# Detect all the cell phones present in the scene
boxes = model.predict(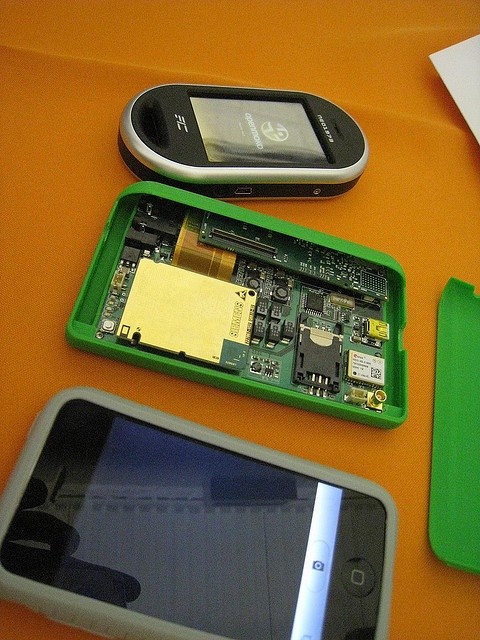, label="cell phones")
[118,83,369,201]
[0,384,398,640]
